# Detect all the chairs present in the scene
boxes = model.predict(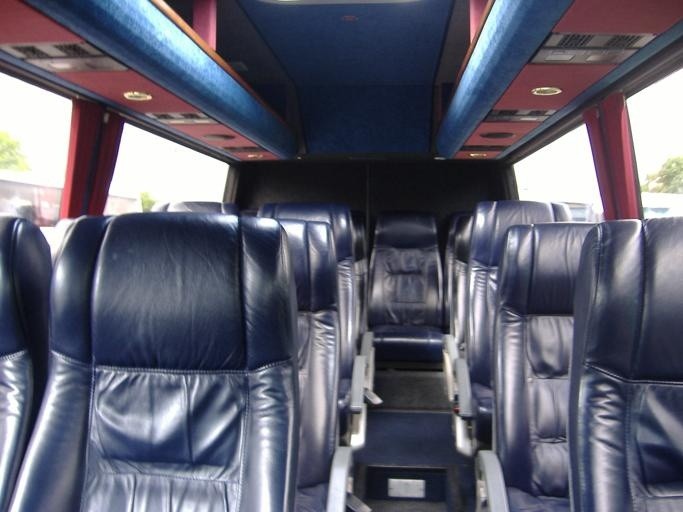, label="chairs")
[368,213,447,364]
